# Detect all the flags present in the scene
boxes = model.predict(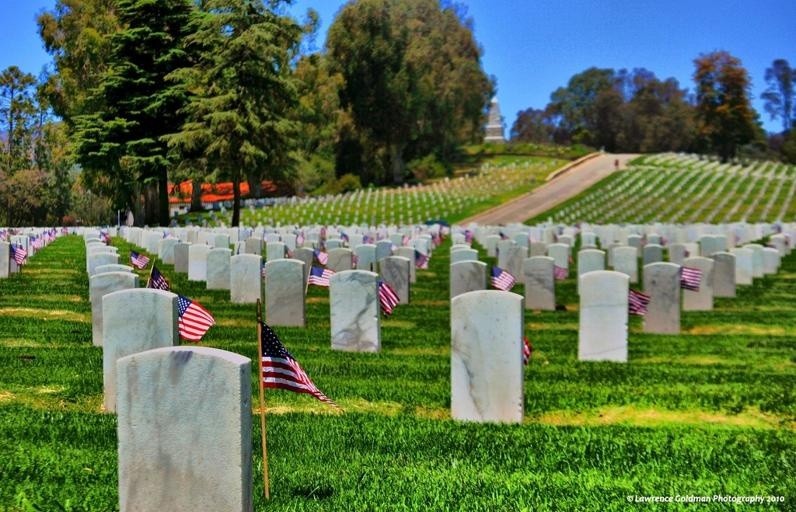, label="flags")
[175,292,215,342]
[628,288,649,318]
[0,221,793,293]
[378,282,399,315]
[523,336,534,364]
[260,321,344,414]
